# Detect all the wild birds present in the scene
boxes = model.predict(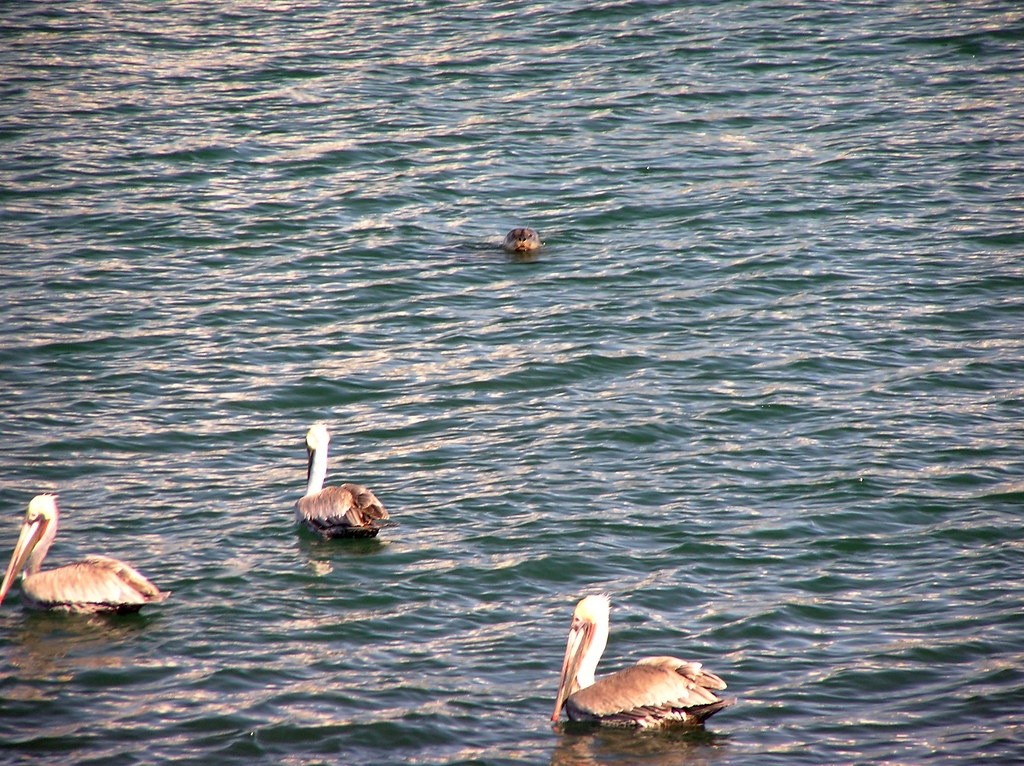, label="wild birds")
[551,592,738,729]
[293,422,401,539]
[0,493,172,614]
